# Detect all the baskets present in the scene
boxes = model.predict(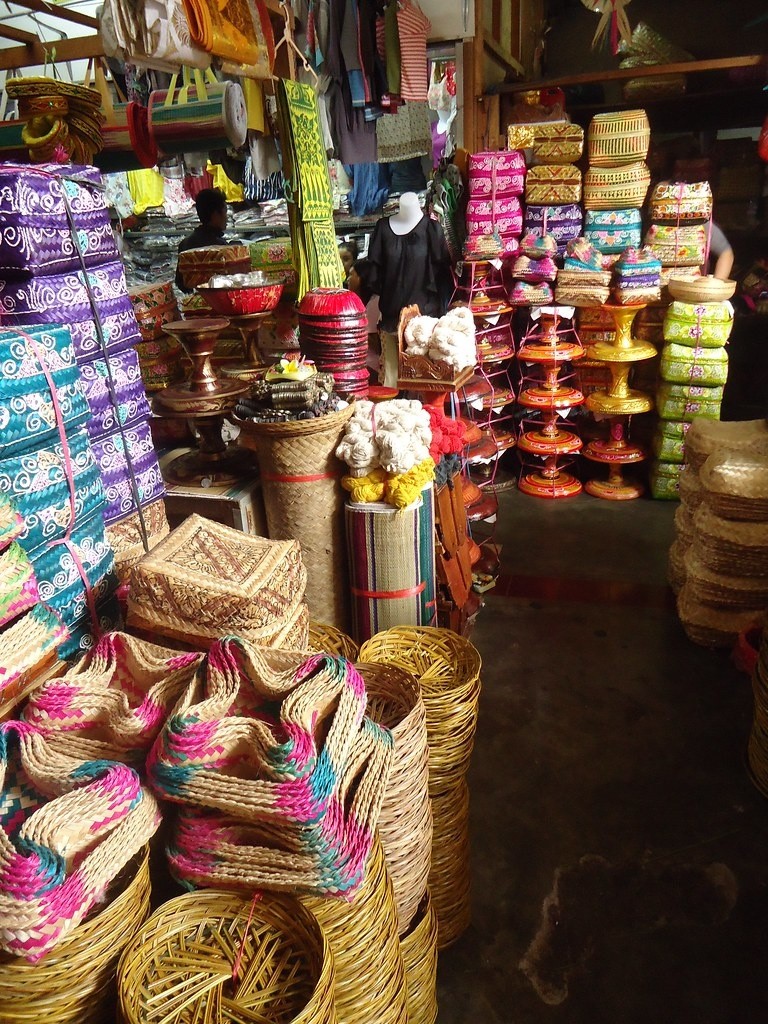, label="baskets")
[0,163,483,1023]
[464,108,767,799]
